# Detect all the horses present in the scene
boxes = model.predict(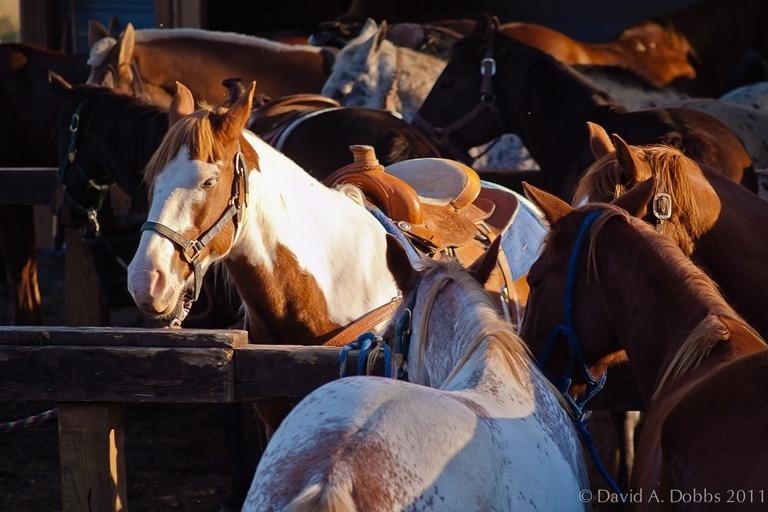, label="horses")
[0,0,768,512]
